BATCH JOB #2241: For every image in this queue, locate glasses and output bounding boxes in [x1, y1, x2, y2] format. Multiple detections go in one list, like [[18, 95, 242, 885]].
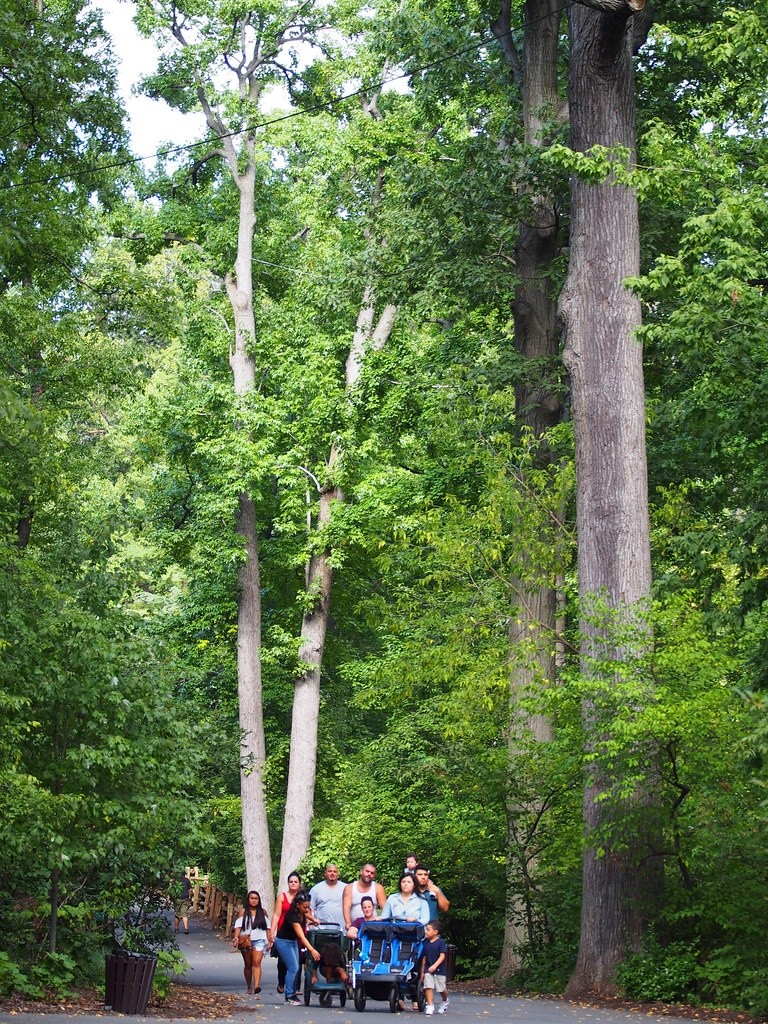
[[297, 894, 311, 901]]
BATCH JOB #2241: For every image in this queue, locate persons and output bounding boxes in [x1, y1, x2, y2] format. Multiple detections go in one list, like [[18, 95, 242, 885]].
[[418, 921, 450, 1015], [232, 890, 273, 994], [269, 854, 451, 1015], [166, 877, 193, 935]]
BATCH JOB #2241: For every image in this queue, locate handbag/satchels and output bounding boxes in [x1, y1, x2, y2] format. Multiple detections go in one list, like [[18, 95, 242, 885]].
[[237, 934, 252, 950]]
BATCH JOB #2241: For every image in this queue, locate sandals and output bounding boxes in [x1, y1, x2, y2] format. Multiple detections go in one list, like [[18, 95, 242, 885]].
[[295, 990, 303, 995], [277, 984, 284, 993]]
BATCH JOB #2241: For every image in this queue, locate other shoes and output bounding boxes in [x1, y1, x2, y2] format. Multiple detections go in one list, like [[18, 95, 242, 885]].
[[429, 892, 438, 900], [398, 1000, 404, 1010], [174, 928, 178, 934], [184, 928, 190, 934], [413, 1002, 418, 1009], [254, 986, 261, 995], [246, 988, 252, 994]]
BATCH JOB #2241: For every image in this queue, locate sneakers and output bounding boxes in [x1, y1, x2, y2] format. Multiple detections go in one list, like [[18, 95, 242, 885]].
[[437, 998, 450, 1014], [284, 994, 302, 1005], [425, 1002, 434, 1014]]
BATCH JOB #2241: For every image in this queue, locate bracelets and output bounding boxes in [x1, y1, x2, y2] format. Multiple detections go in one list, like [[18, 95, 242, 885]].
[[233, 937, 239, 941]]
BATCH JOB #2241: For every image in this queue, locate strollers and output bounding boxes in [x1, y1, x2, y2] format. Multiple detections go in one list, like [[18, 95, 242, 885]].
[[350, 917, 427, 1014], [304, 922, 352, 1009]]
